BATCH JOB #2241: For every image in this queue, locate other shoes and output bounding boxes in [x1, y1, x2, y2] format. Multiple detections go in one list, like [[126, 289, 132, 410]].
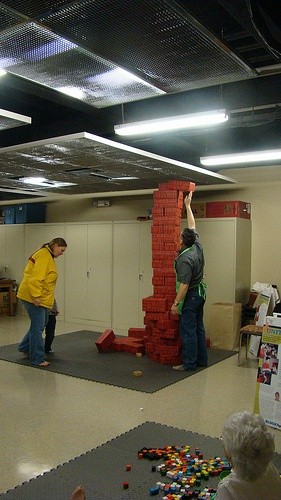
[[173, 365, 185, 370]]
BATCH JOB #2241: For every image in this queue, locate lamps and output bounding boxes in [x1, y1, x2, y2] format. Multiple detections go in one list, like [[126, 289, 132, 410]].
[[112, 109, 281, 167]]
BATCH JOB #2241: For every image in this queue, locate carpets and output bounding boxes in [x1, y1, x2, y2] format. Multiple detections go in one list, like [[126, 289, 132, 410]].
[[0, 421, 281, 500], [3, 330, 239, 394]]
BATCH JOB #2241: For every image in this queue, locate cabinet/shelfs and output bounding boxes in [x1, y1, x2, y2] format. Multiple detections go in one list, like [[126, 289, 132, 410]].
[[0, 216, 253, 328]]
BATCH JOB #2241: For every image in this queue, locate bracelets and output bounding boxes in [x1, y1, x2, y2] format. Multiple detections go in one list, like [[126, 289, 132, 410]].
[[173, 301, 179, 306]]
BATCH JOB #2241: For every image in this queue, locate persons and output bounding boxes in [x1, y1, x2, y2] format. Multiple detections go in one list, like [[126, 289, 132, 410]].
[[45, 300, 59, 354], [170, 191, 208, 372], [16, 238, 67, 367], [216, 411, 281, 500]]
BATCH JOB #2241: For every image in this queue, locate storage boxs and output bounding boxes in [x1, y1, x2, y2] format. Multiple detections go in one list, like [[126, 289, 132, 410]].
[[94, 180, 250, 366], [253, 315, 281, 428]]
